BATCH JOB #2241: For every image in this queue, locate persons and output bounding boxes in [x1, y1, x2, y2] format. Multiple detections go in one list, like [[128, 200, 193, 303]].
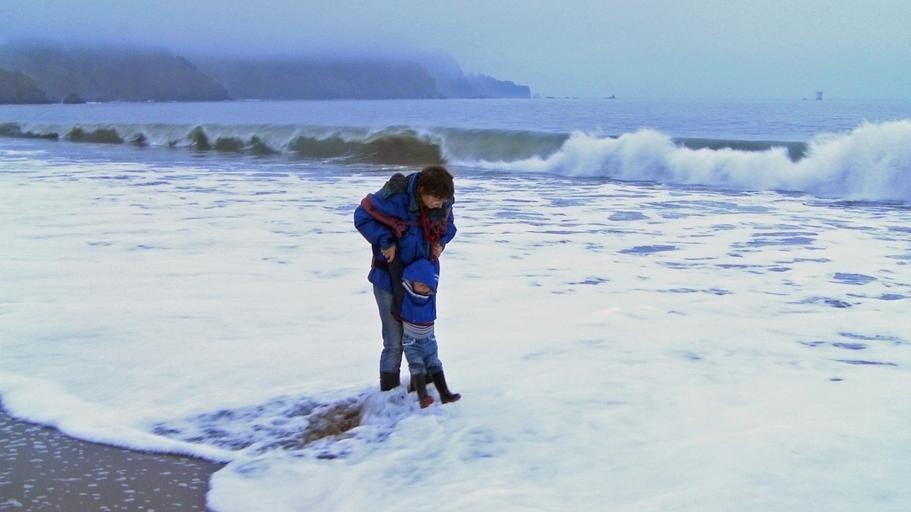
[[387, 245, 461, 409], [354, 167, 457, 391]]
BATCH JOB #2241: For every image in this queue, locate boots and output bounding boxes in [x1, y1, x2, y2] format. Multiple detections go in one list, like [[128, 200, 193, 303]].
[[432, 370, 461, 404], [413, 373, 434, 409]]
